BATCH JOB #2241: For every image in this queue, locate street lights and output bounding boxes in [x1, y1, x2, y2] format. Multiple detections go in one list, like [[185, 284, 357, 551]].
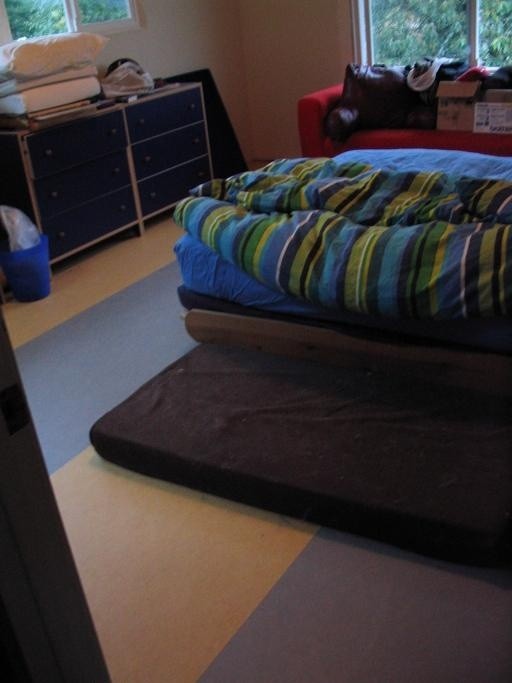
[[342, 64, 404, 112]]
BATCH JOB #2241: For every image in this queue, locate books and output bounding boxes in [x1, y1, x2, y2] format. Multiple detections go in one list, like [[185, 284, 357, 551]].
[[435, 79, 511, 134]]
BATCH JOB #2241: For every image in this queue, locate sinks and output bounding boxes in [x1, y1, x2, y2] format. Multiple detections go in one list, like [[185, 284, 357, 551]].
[[296, 66, 510, 156]]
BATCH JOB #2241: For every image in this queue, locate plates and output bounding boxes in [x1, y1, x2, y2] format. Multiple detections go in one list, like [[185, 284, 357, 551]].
[[0, 78, 214, 274]]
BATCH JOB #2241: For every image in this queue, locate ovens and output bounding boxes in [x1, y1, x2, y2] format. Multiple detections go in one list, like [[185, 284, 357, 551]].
[[171, 148, 511, 380]]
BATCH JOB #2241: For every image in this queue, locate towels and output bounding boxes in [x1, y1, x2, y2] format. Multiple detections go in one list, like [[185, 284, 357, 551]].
[[0, 234, 51, 303]]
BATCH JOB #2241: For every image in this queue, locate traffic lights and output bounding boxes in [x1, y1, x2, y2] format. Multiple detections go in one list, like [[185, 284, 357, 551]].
[[0, 32, 111, 80]]
